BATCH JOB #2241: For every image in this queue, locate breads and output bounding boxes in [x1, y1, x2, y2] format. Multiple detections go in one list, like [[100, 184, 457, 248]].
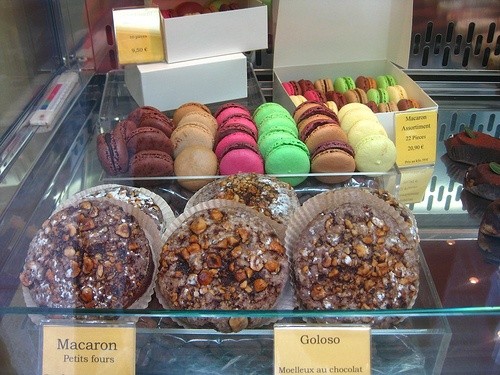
[[444, 126, 500, 250]]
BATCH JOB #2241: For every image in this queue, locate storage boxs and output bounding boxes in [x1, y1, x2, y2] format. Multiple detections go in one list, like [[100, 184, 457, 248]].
[[124, 52, 247, 113], [148, 0, 268, 64], [272, 0, 438, 170]]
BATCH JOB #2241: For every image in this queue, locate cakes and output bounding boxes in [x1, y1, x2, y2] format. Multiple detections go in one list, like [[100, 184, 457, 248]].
[[369, 190, 414, 226], [156, 207, 288, 333], [208, 172, 290, 223], [78, 186, 166, 233], [18, 200, 154, 320], [291, 203, 420, 328]]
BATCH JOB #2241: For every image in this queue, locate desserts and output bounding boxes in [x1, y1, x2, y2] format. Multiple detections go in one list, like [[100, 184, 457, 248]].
[[98, 102, 393, 190], [281, 75, 422, 114]]
